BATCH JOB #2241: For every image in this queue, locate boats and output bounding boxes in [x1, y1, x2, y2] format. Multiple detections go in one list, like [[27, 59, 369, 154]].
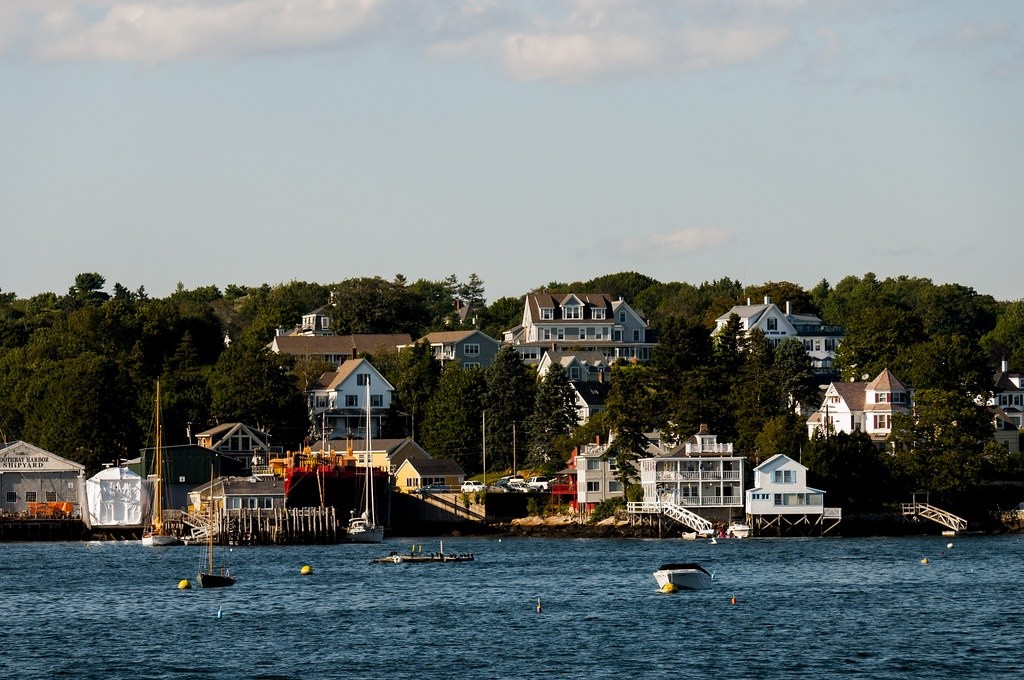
[[725, 524, 752, 540], [370, 554, 475, 564], [683, 531, 707, 539], [652, 562, 716, 592]]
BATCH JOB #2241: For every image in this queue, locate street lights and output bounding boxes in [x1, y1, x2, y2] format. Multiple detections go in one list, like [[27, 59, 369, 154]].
[[483, 408, 492, 486]]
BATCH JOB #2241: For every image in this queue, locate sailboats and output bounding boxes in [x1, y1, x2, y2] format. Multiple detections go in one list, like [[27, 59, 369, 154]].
[[346, 378, 384, 542], [195, 464, 235, 588], [143, 382, 178, 547]]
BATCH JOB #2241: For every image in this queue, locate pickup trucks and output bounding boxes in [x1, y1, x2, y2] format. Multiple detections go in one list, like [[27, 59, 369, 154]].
[[528, 476, 554, 492]]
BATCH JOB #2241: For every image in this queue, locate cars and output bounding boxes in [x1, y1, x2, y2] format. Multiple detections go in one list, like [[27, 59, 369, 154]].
[[461, 480, 487, 493], [508, 482, 531, 493], [416, 482, 451, 493]]
